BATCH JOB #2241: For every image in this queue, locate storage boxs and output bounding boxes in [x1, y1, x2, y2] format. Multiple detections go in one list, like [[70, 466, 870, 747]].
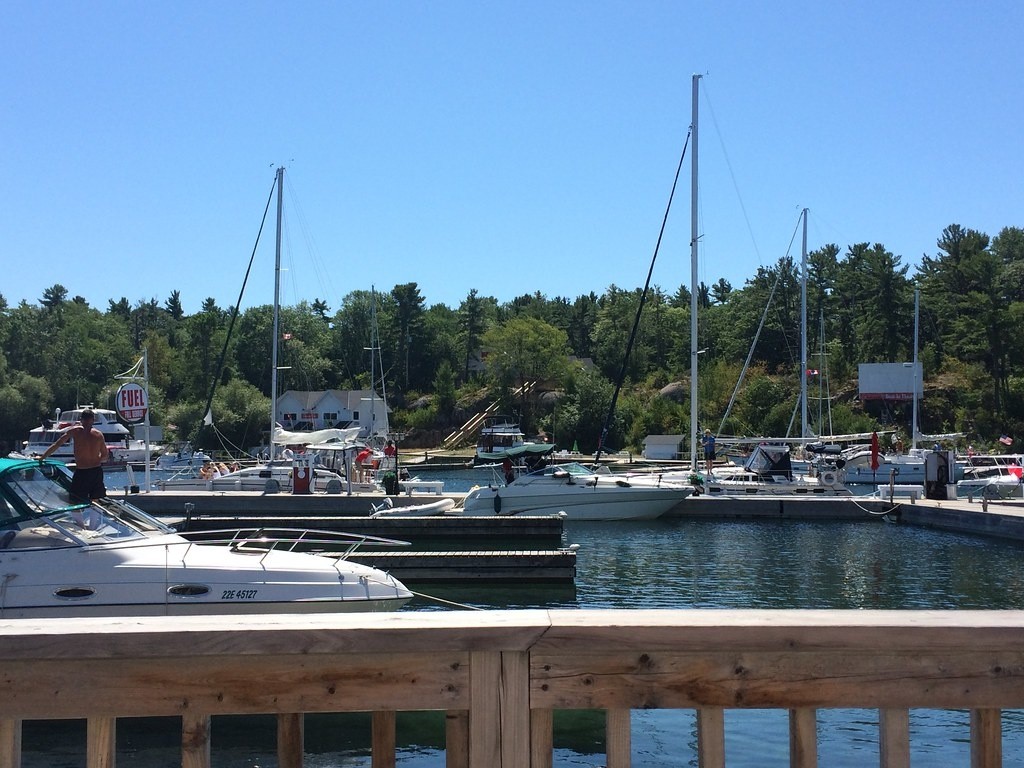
[[878, 484, 924, 500]]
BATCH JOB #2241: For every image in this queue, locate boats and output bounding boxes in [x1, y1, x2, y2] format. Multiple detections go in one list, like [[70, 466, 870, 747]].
[[457, 444, 691, 521], [0, 458, 415, 611], [22, 404, 163, 469]]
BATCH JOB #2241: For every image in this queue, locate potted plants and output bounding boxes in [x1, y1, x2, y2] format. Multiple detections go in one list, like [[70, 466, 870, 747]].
[[687, 474, 705, 496], [380, 470, 397, 495]]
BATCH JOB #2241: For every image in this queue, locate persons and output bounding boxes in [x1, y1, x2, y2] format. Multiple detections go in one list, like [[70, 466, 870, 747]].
[[897, 436, 904, 458], [933, 440, 942, 453], [526, 450, 548, 476], [968, 442, 974, 459], [33, 410, 110, 531], [355, 439, 400, 488], [702, 428, 717, 476], [504, 454, 517, 486], [198, 459, 240, 483]]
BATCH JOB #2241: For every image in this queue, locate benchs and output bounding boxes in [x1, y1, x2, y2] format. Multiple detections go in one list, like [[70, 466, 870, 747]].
[[402, 481, 445, 496]]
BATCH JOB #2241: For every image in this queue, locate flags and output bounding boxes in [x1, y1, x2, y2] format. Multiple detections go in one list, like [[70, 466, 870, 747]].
[[204, 408, 213, 426], [807, 368, 821, 376], [1000, 433, 1015, 445]]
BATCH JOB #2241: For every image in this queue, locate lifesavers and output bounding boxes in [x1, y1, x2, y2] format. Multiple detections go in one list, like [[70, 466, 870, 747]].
[[821, 471, 837, 486]]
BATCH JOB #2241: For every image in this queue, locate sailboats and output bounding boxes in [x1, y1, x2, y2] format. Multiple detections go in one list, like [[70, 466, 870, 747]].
[[104, 159, 466, 516], [584, 72, 829, 495], [701, 199, 1024, 495]]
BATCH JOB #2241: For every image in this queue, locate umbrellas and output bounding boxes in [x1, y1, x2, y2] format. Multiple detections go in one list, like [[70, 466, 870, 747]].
[[871, 429, 881, 494]]
